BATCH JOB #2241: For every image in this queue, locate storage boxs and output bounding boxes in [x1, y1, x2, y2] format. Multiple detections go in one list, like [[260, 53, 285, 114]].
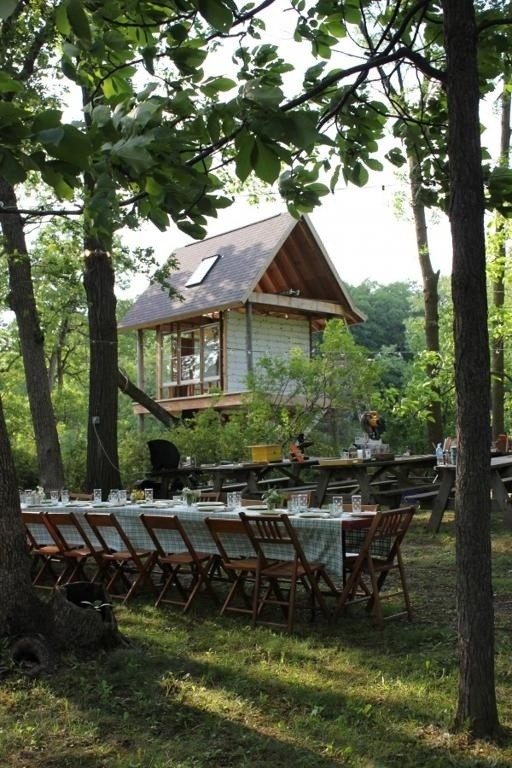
[[247, 443, 283, 462]]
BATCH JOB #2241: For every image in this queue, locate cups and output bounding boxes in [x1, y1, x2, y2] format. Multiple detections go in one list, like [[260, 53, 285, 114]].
[[332, 495, 362, 516], [227, 491, 242, 509], [287, 494, 308, 513], [26, 488, 182, 508], [340, 449, 371, 460]]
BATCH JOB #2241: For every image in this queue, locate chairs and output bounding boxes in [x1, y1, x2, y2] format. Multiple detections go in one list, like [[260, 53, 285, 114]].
[[20, 483, 417, 631], [136, 436, 181, 496]]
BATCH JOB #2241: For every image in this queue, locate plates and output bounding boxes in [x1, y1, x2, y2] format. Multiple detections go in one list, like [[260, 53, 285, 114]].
[[197, 502, 226, 512], [301, 512, 329, 518], [247, 502, 283, 515], [350, 512, 379, 516]]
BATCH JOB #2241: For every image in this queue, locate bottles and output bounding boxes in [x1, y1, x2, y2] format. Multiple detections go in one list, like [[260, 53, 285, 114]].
[[436, 443, 449, 465]]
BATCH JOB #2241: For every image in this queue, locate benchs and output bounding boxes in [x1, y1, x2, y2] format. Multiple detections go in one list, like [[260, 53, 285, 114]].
[[148, 456, 510, 533]]
